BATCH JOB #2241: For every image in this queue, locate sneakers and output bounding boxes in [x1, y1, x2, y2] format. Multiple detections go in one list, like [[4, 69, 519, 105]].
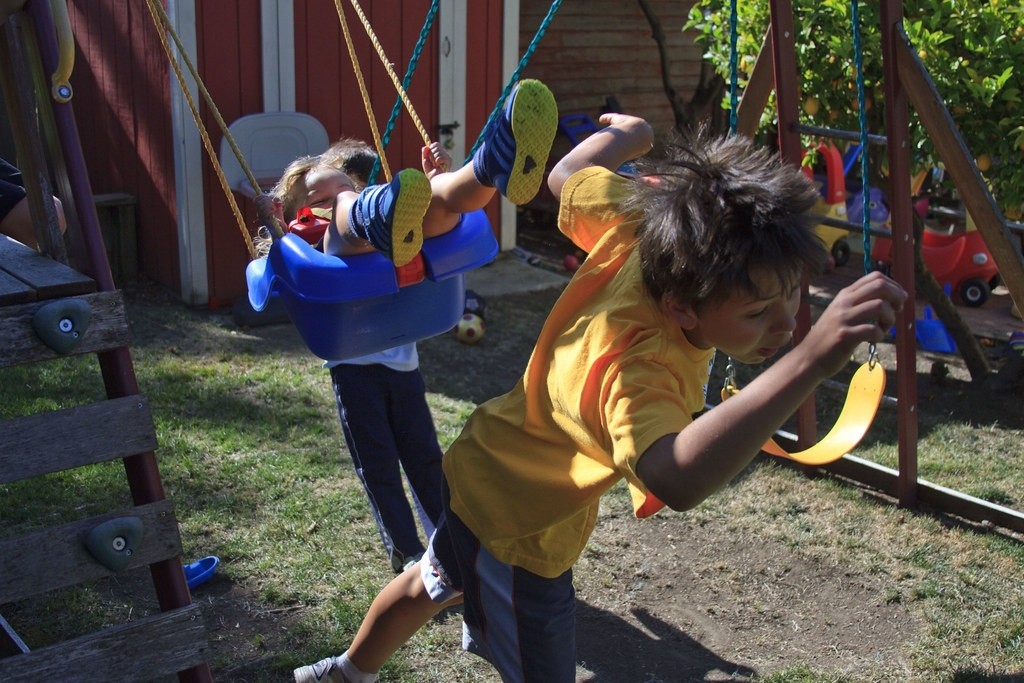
[[361, 168, 433, 269], [294, 654, 346, 683], [483, 79, 559, 206]]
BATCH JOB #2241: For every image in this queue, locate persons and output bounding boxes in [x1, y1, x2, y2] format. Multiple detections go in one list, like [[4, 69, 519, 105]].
[[0, 148, 66, 252], [322, 137, 453, 575], [292, 113, 907, 683], [252, 78, 557, 267]]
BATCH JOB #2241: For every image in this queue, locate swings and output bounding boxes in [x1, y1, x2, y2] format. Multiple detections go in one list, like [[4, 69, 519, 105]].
[[145, 0, 501, 362], [719, 0, 888, 466]]
